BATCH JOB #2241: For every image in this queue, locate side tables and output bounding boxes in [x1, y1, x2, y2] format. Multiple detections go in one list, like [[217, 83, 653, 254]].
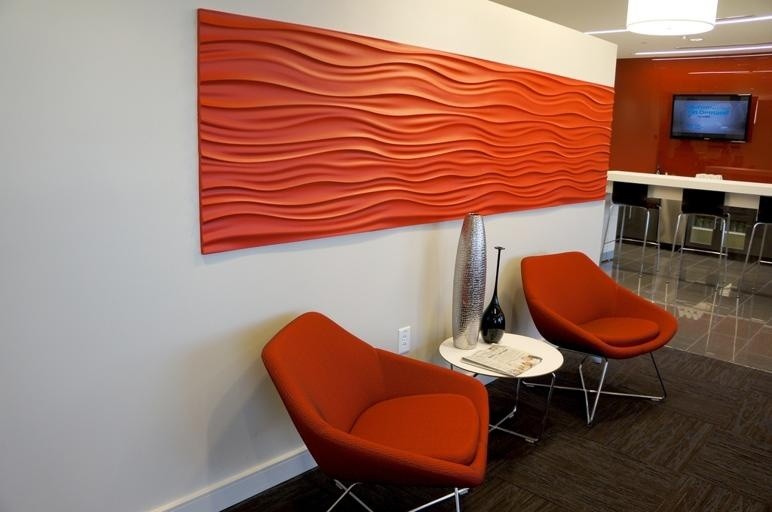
[[438, 331, 565, 442]]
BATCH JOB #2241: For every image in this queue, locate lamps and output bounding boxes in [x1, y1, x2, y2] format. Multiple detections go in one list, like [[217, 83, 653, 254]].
[[625, 0, 719, 37]]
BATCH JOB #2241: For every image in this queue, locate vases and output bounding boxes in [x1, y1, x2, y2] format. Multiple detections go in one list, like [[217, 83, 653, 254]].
[[481, 247, 506, 344], [452, 213, 487, 350]]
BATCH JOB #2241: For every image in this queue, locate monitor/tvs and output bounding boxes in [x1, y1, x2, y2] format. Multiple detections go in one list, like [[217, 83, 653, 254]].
[[669, 93, 752, 143]]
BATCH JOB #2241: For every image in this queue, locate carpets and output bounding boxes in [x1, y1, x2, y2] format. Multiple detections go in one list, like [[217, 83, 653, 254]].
[[216, 346, 772, 512]]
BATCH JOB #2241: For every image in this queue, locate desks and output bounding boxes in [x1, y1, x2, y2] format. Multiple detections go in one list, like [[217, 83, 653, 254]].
[[600, 170, 772, 263]]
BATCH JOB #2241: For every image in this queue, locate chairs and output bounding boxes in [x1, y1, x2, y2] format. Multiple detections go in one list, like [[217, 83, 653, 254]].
[[520, 251, 678, 425], [261, 312, 490, 512], [736, 196, 772, 299], [600, 181, 662, 278], [666, 188, 731, 292]]
